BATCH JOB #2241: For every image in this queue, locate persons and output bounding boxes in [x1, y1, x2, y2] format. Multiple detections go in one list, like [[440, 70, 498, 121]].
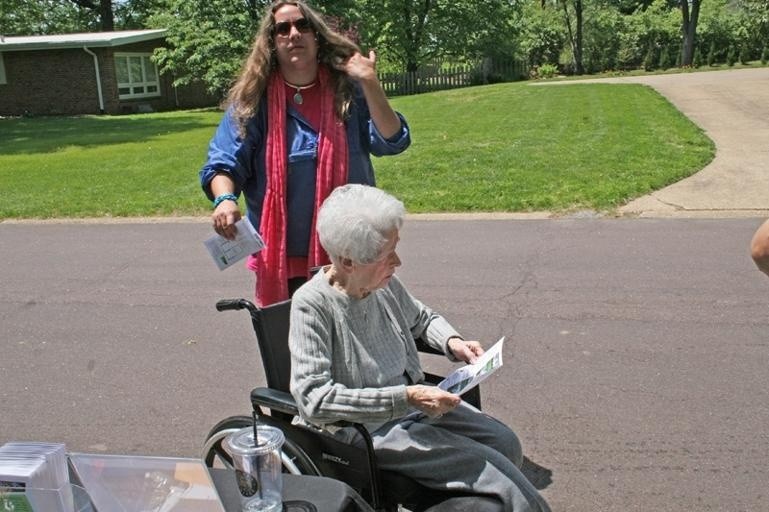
[[286, 182, 550, 510], [747, 218, 769, 281], [201, 1, 410, 307]]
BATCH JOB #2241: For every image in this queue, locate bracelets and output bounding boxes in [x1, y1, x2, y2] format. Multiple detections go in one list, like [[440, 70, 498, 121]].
[[212, 193, 238, 208]]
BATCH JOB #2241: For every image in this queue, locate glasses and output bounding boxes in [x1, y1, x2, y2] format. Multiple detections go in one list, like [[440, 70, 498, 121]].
[[273, 16, 313, 37]]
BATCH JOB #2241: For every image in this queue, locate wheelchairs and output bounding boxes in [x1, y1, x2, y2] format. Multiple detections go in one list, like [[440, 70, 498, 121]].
[[200, 265, 482, 512]]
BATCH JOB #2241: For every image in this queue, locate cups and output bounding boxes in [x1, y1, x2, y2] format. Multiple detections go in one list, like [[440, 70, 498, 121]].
[[225, 423, 288, 512]]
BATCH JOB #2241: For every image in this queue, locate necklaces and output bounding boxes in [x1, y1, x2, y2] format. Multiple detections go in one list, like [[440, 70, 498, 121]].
[[286, 82, 318, 104]]
